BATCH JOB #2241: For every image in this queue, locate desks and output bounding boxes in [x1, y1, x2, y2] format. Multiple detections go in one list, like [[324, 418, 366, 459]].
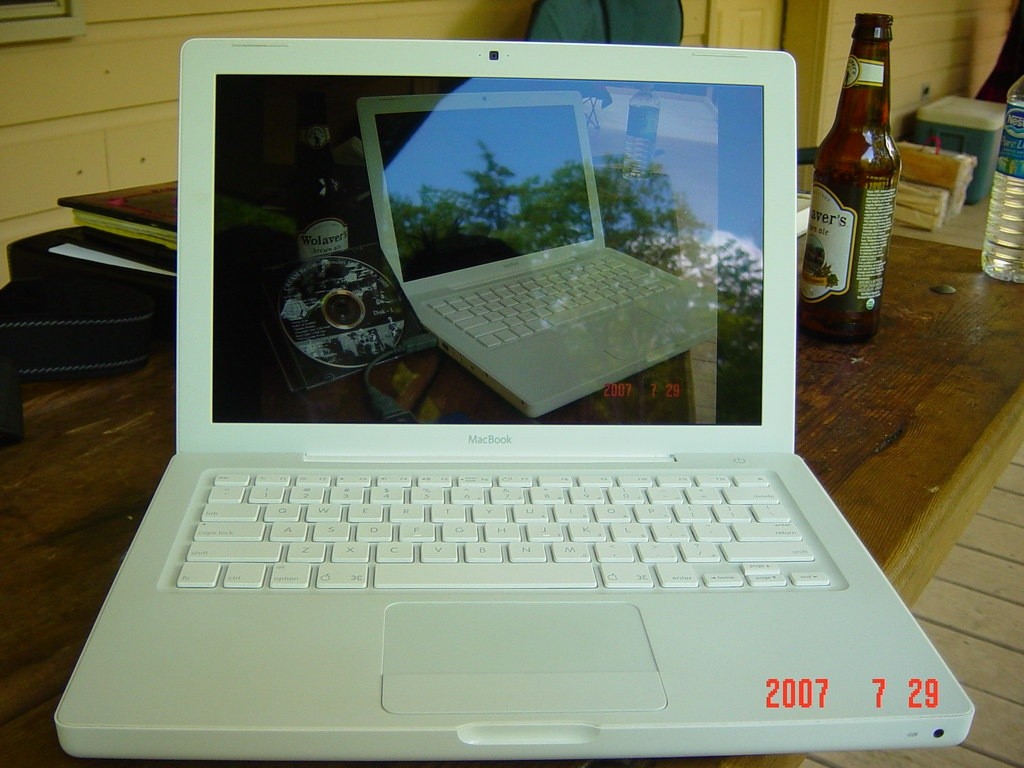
[[1, 236, 1024, 768]]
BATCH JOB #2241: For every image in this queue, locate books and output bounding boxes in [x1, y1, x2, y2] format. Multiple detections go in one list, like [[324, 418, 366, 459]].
[[57, 180, 178, 251]]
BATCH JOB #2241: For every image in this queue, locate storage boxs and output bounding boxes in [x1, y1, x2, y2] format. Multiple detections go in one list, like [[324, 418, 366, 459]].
[[892, 95, 1007, 232]]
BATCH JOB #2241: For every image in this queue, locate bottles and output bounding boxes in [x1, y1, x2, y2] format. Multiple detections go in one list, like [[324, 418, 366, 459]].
[[296, 91, 351, 259], [623, 85, 660, 181], [798, 13, 901, 343], [981, 72, 1024, 284]]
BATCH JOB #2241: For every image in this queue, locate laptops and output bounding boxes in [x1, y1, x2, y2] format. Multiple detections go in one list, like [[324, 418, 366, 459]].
[[55, 37, 976, 763], [357, 91, 719, 419]]
